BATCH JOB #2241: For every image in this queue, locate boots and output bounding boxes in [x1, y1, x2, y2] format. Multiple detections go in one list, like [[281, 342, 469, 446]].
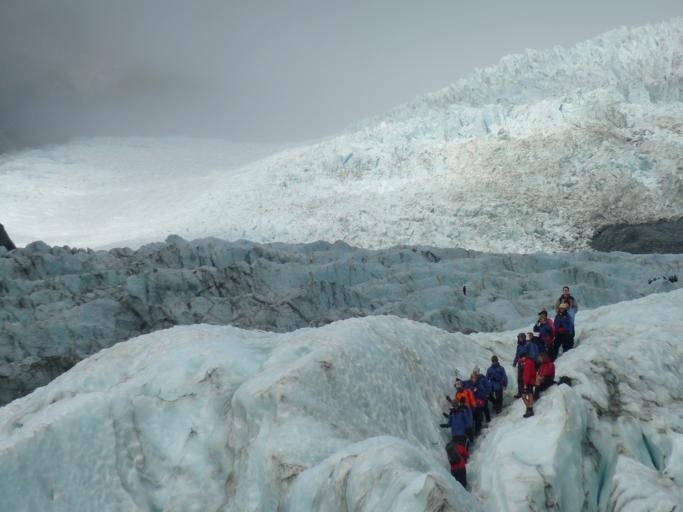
[[524, 407, 534, 417]]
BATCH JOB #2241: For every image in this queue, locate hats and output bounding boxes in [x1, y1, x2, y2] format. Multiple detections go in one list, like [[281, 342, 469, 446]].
[[559, 304, 567, 311], [473, 368, 480, 376], [455, 381, 462, 388], [452, 399, 458, 406], [539, 311, 547, 315]]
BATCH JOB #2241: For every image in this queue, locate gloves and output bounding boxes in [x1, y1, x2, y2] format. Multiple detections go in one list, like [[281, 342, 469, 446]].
[[440, 424, 447, 428], [443, 412, 448, 418], [446, 396, 451, 402]]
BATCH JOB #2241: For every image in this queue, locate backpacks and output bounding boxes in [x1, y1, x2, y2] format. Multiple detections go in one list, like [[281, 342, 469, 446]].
[[445, 443, 461, 465]]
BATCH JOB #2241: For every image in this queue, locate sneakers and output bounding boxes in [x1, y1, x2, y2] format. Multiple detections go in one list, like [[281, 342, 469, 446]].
[[514, 392, 521, 398], [474, 402, 502, 435]]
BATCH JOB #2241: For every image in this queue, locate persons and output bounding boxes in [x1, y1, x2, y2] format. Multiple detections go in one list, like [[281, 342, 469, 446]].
[[437, 287, 577, 488]]
